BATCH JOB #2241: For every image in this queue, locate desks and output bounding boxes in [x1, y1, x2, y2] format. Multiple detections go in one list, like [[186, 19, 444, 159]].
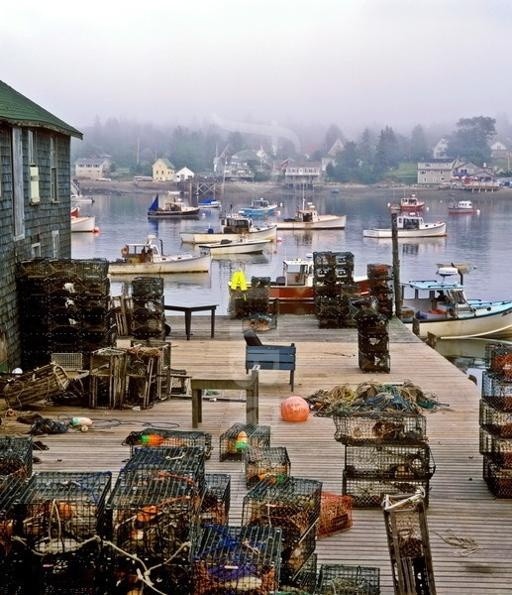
[[164, 305, 218, 340]]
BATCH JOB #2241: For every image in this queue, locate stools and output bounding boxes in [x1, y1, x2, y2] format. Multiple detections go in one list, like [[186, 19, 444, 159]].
[[191, 365, 260, 427]]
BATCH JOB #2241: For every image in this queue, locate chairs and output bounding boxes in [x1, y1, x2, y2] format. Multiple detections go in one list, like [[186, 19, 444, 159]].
[[245, 343, 296, 392]]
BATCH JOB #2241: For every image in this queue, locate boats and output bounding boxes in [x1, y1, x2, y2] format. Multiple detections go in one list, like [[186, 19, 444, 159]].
[[70, 188, 96, 234]]
